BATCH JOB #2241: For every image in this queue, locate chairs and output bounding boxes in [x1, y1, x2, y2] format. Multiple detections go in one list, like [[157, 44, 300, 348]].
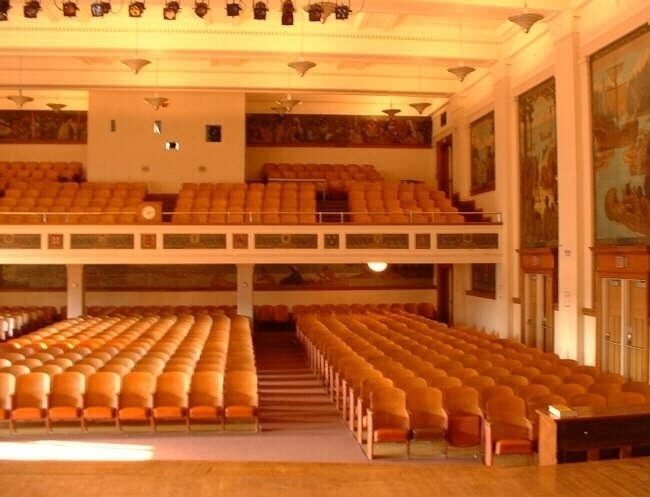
[[0, 161, 147, 223], [347, 179, 464, 226], [170, 180, 317, 226], [0, 302, 262, 435], [292, 301, 650, 464], [252, 304, 295, 332], [262, 162, 384, 198]]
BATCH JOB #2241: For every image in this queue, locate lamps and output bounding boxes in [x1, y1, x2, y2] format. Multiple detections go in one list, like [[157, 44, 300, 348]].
[[225, 2, 241, 15], [271, 104, 288, 119], [281, 94, 300, 112], [280, 1, 295, 25], [121, 57, 151, 75], [205, 124, 222, 143], [162, 1, 181, 20], [448, 62, 476, 83], [381, 101, 401, 119], [151, 103, 169, 111], [288, 54, 317, 78], [192, 2, 210, 17], [144, 97, 168, 104], [46, 103, 67, 112], [333, 1, 351, 19], [253, 1, 268, 20], [62, 2, 79, 17], [128, 2, 147, 17], [508, 1, 544, 33], [90, 1, 113, 17], [409, 102, 432, 115], [24, 1, 41, 16], [4, 56, 34, 107], [307, 1, 323, 22]]
